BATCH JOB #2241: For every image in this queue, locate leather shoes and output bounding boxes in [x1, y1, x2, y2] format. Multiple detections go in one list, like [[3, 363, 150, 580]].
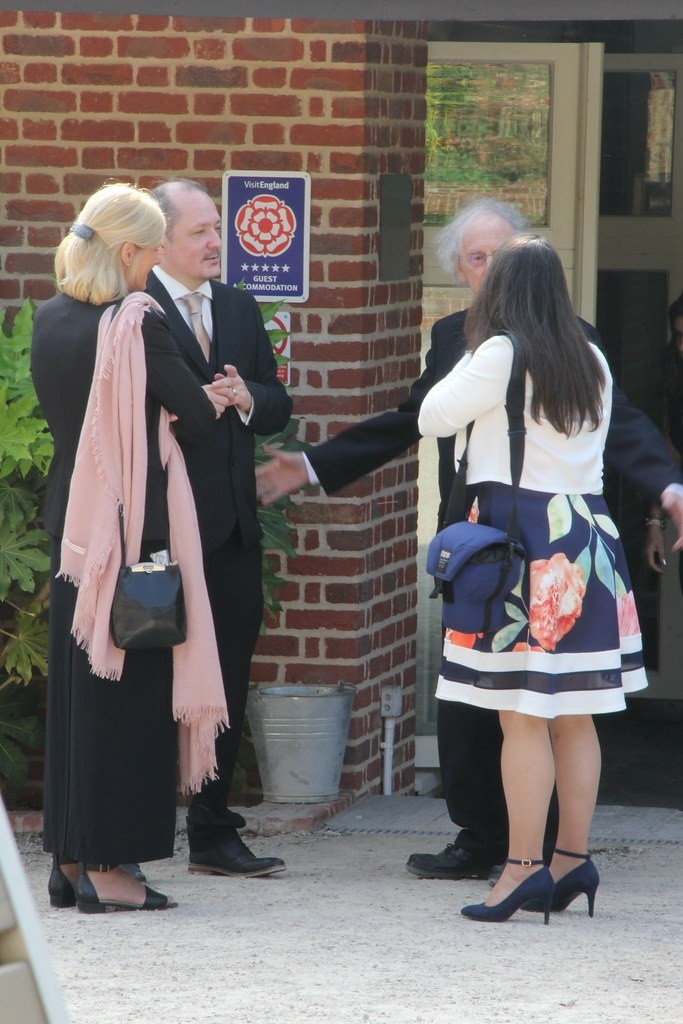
[[187, 834, 286, 878], [120, 863, 147, 882], [406, 830, 491, 879]]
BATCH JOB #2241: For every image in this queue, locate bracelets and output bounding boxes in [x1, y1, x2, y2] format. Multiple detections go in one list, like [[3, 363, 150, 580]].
[[644, 512, 666, 529]]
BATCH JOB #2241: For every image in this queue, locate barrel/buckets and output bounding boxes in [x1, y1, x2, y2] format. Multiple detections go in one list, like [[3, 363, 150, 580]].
[[246, 680, 357, 803]]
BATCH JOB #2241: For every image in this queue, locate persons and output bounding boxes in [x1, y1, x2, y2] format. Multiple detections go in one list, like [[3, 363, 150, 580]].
[[256, 200, 683, 881], [418, 235, 648, 925], [153, 176, 295, 879], [644, 294, 682, 592], [31, 183, 219, 913]]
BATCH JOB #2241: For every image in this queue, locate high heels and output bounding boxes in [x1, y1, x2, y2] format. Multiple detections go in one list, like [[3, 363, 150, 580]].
[[522, 849, 600, 918], [460, 860, 554, 926]]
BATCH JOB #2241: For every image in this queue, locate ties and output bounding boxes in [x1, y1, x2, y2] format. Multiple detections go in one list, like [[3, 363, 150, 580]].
[[181, 294, 210, 363]]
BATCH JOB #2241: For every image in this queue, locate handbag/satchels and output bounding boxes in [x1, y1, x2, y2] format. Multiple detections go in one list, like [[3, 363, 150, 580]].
[[110, 562, 187, 650], [427, 522, 524, 633]]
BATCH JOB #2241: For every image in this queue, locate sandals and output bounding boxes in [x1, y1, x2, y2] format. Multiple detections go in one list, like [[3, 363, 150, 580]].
[[77, 861, 178, 910], [49, 854, 77, 908]]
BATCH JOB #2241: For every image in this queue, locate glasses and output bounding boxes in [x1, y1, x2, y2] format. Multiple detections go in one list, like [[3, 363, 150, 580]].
[[467, 251, 501, 267]]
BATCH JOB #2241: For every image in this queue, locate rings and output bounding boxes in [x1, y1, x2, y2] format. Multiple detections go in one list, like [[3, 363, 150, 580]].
[[231, 388, 236, 394]]
